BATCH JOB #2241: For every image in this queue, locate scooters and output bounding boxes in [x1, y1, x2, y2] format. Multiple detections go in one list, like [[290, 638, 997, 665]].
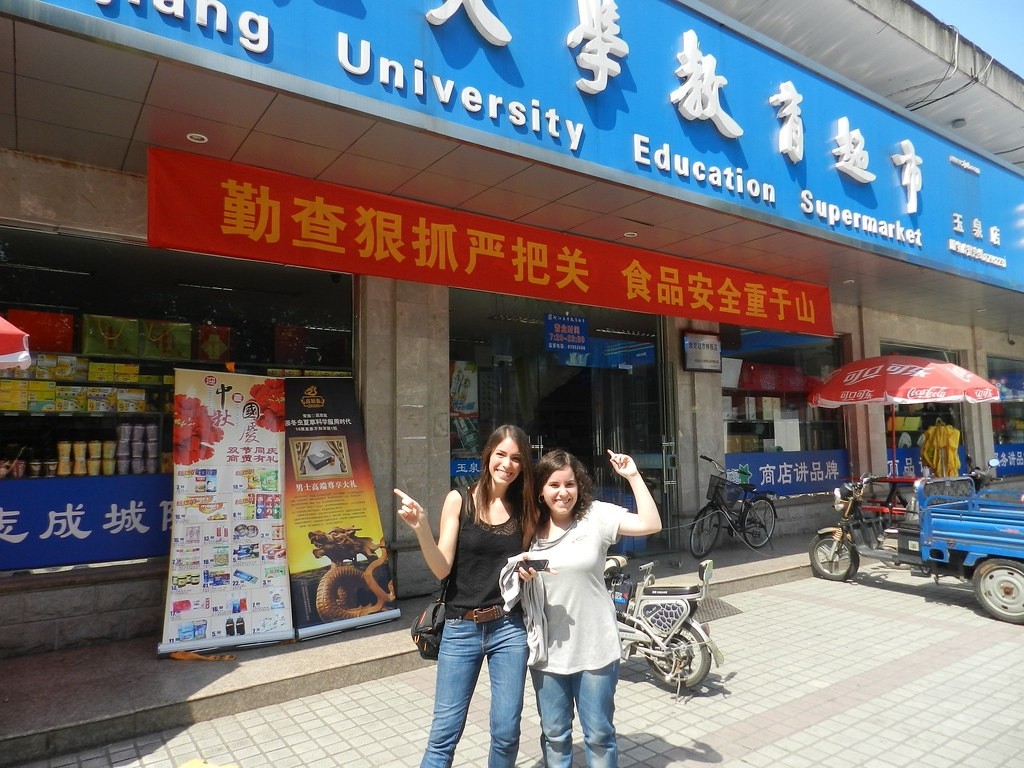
[[603, 554, 726, 703]]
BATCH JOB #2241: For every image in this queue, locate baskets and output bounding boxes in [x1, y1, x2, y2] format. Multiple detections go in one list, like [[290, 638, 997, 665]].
[[706, 474, 744, 509]]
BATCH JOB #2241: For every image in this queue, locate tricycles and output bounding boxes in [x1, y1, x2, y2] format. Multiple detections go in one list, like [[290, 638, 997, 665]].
[[809, 461, 1024, 627]]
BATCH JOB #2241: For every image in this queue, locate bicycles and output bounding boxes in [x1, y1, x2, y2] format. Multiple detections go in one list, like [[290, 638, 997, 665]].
[[690, 454, 779, 559]]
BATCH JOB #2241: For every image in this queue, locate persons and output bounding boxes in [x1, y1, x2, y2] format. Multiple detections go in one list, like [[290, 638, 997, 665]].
[[519, 448, 662, 768], [391, 425, 537, 768]]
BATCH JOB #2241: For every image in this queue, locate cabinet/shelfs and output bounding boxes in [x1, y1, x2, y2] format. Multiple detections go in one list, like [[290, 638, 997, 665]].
[[722, 386, 838, 436], [992, 401, 1024, 432], [0, 350, 353, 474], [885, 410, 961, 438]]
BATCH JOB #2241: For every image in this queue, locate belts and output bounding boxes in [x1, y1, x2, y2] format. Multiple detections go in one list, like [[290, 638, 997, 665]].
[[461, 604, 522, 624]]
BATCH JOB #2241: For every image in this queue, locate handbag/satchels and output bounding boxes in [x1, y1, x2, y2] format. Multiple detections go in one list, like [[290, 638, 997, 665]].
[[410, 603, 446, 661]]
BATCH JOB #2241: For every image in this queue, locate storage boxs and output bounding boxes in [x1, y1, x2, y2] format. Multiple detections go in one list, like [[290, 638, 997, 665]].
[[114, 374, 139, 382], [138, 375, 163, 384], [162, 389, 174, 403], [193, 325, 231, 362], [144, 391, 163, 402], [722, 358, 829, 454], [0, 353, 118, 413], [138, 318, 191, 359], [163, 375, 174, 384], [117, 400, 149, 412], [5, 309, 74, 353], [118, 388, 149, 400], [76, 314, 139, 357], [274, 325, 306, 364], [115, 363, 140, 374], [163, 403, 174, 413], [148, 402, 163, 412]]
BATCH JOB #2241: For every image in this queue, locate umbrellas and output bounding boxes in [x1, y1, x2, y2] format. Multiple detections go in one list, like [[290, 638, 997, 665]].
[[806, 353, 1001, 477]]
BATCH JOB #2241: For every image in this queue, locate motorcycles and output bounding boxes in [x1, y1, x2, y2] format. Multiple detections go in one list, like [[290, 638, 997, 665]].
[[961, 453, 1004, 496]]
